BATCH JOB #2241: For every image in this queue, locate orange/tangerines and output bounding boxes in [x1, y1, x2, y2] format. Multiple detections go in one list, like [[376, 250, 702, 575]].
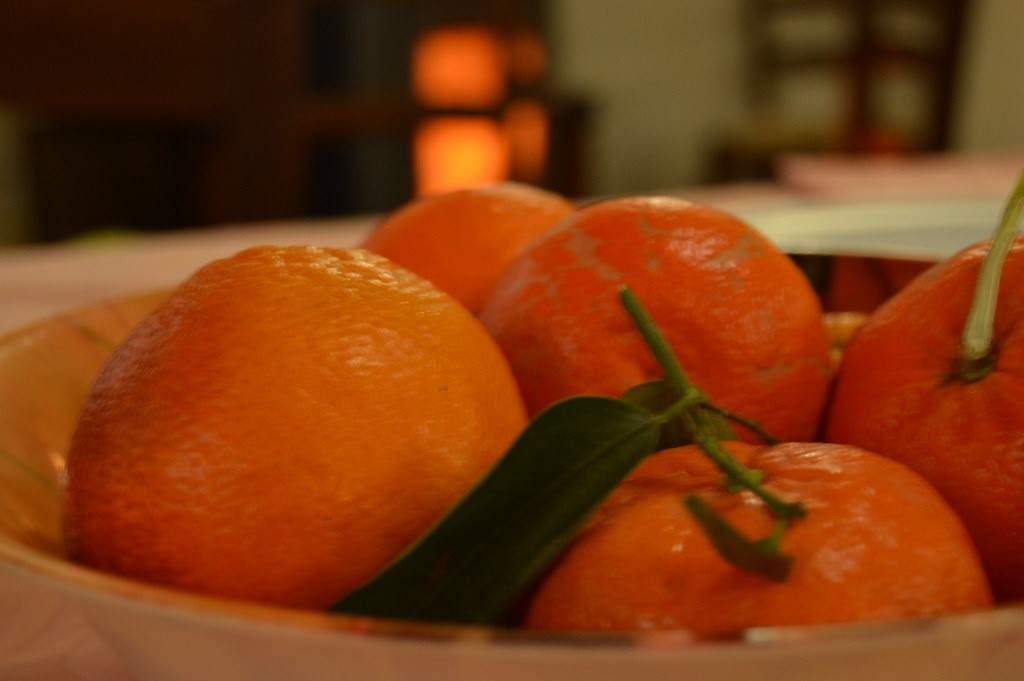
[[62, 182, 1024, 627]]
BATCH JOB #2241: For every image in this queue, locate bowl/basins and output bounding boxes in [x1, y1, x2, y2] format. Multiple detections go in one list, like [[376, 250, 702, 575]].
[[0, 252, 1024, 681]]
